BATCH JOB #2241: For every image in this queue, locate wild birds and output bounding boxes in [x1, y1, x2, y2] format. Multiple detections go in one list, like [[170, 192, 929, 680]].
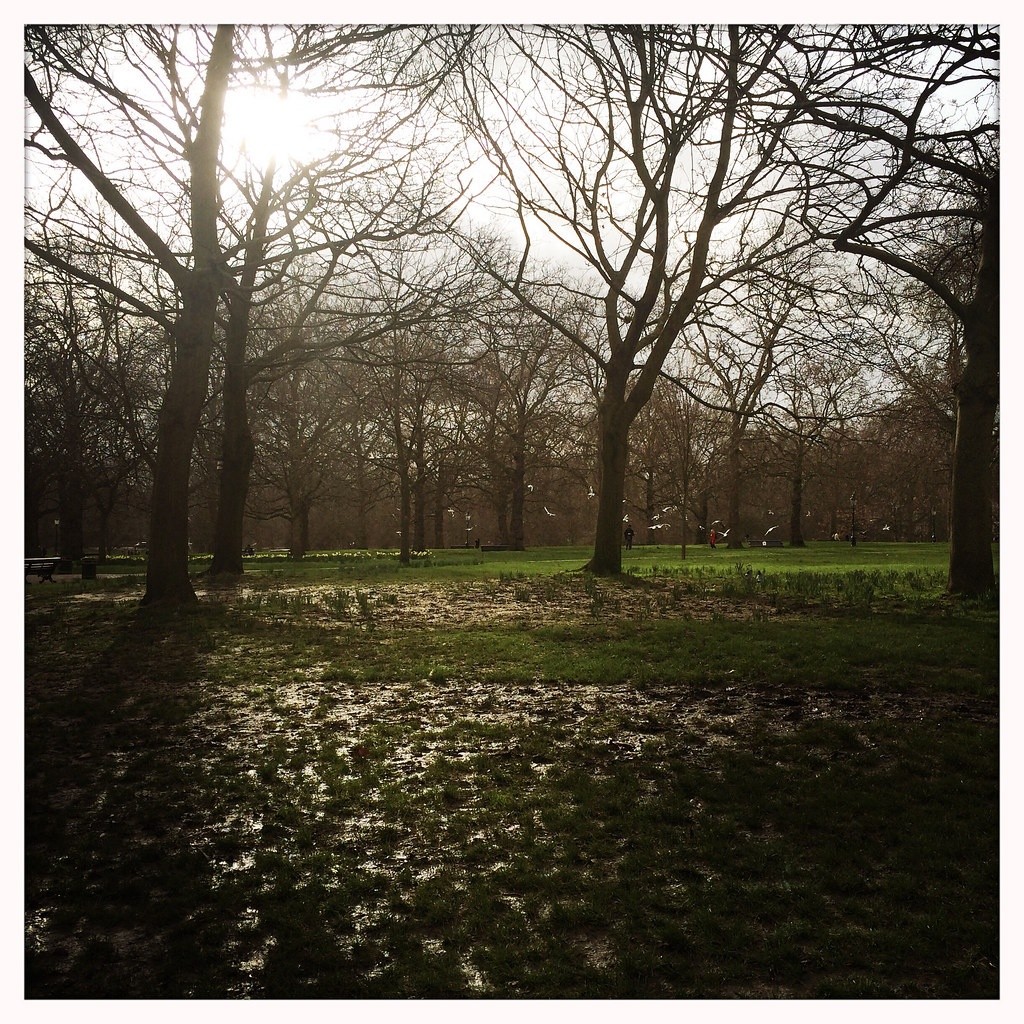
[[882, 524, 890, 530], [806, 510, 811, 516], [768, 510, 774, 515], [648, 506, 671, 529], [623, 514, 629, 523], [587, 485, 595, 498], [718, 529, 731, 538], [544, 507, 556, 516], [764, 525, 779, 535]]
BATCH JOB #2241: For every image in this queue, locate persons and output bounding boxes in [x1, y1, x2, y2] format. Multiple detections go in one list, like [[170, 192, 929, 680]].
[[475, 538, 479, 548], [624, 525, 634, 550], [245, 545, 253, 556], [709, 529, 716, 548]]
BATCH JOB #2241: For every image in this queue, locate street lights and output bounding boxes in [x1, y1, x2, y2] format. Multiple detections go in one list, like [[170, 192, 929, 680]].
[[465, 512, 471, 548], [850, 491, 857, 547], [54, 515, 61, 557], [931, 505, 936, 543]]
